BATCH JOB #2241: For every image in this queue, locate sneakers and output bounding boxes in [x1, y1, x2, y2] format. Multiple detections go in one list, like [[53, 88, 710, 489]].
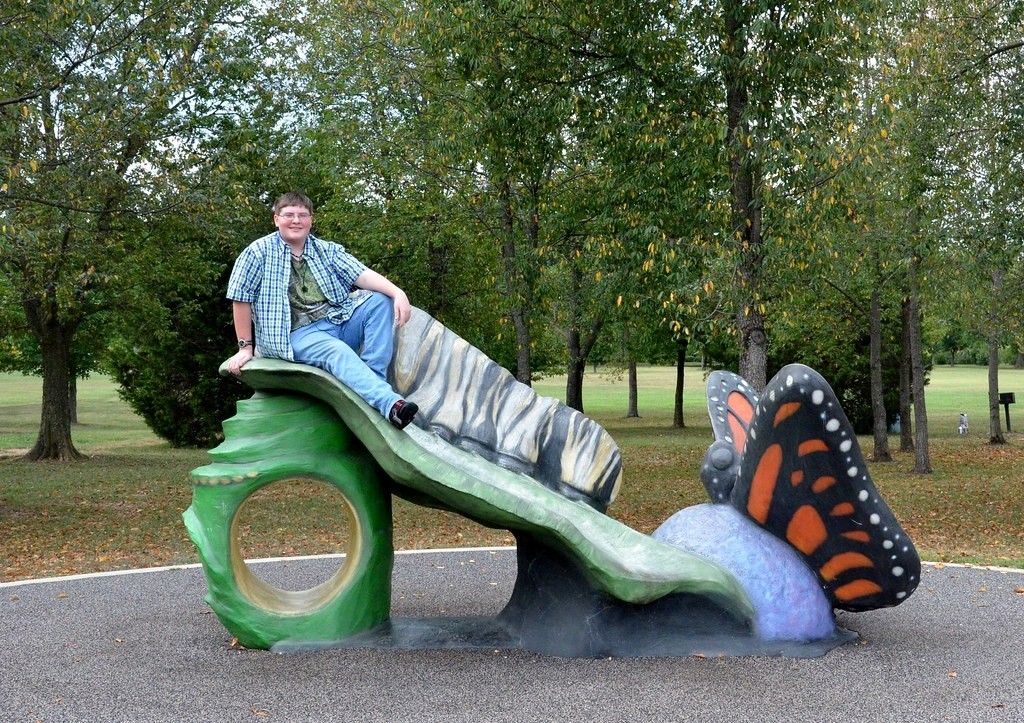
[[389, 400, 419, 430]]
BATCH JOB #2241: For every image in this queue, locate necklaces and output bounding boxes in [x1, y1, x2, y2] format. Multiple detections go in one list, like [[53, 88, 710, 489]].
[[290, 251, 313, 293]]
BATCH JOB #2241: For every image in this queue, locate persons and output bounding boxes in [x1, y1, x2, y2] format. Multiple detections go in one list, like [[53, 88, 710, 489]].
[[228, 192, 419, 430]]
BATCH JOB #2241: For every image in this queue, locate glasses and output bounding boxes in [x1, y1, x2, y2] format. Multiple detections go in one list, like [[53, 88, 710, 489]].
[[277, 212, 312, 221]]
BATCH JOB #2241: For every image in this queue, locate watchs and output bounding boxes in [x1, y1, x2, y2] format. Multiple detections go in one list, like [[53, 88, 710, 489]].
[[237, 338, 253, 348]]
